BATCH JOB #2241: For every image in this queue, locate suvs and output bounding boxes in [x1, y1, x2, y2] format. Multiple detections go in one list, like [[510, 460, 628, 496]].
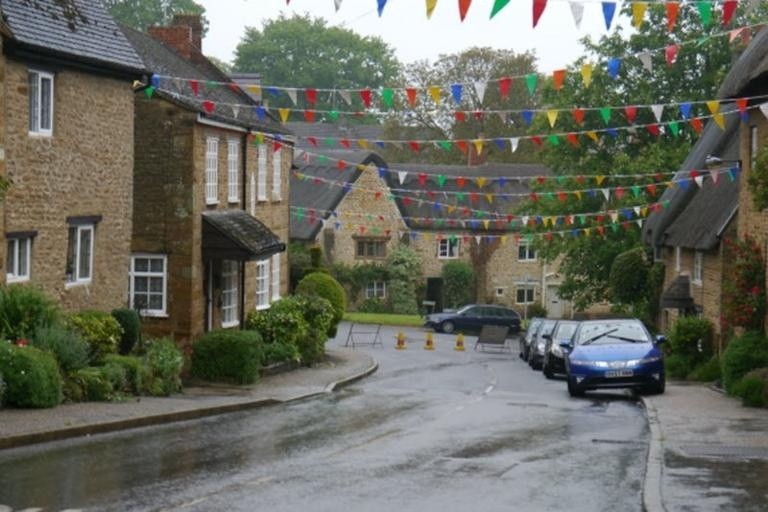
[[424, 305, 521, 340]]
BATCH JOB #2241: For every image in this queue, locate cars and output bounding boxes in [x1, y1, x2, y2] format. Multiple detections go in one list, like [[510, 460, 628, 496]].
[[517, 315, 668, 398]]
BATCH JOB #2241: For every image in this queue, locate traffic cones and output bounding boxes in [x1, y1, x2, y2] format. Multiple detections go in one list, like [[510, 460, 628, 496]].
[[395, 330, 408, 350], [452, 332, 464, 351], [424, 332, 436, 350]]
